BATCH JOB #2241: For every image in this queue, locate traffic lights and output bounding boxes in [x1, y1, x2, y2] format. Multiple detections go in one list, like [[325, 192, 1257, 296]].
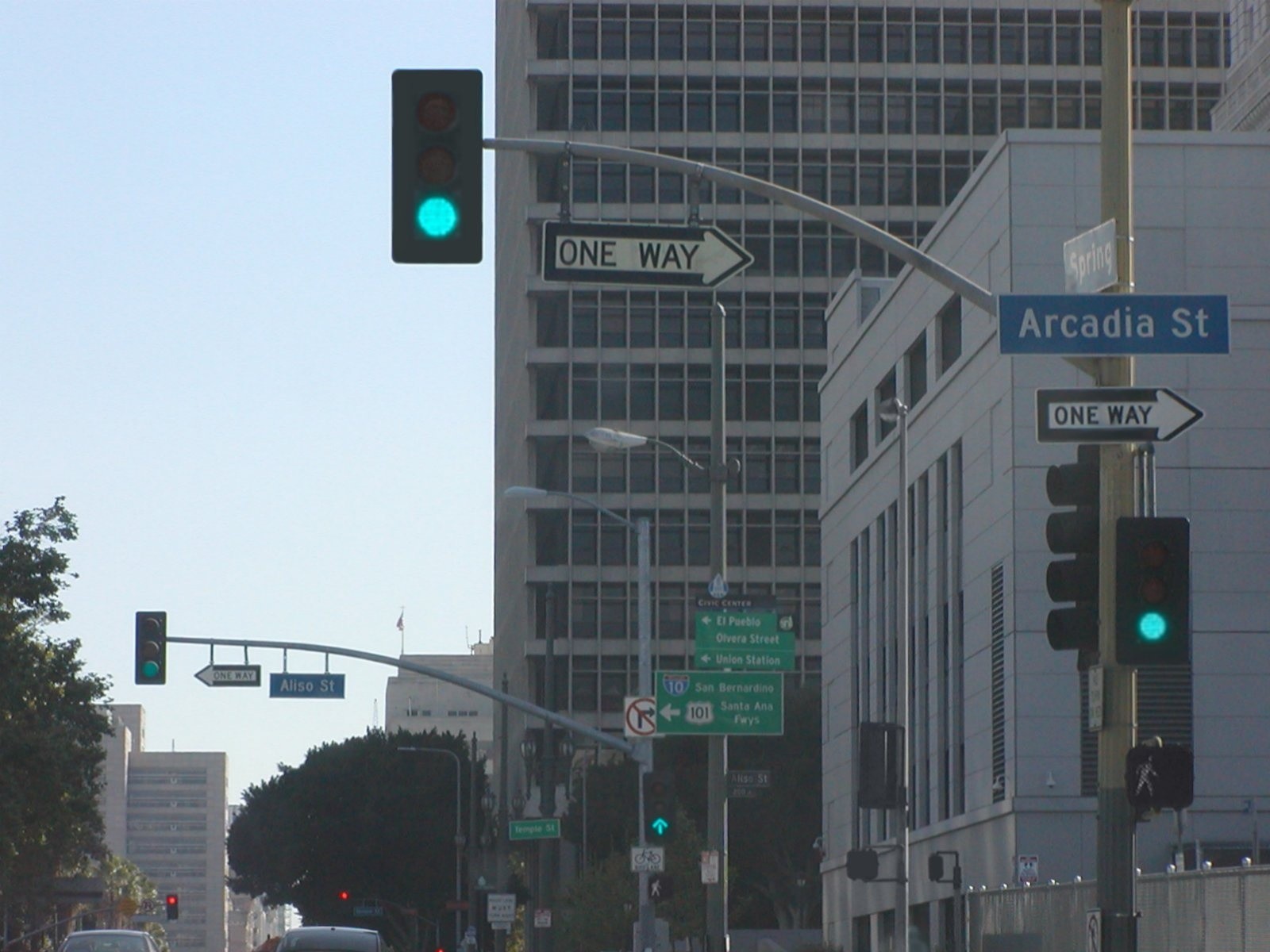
[[1043, 436, 1101, 669], [335, 888, 349, 910], [134, 611, 167, 685], [390, 68, 484, 265], [165, 894, 179, 920], [1125, 735, 1195, 814], [1113, 516, 1193, 668]]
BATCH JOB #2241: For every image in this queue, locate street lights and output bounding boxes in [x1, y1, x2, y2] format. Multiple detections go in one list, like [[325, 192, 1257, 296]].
[[583, 425, 730, 952], [503, 482, 656, 952], [397, 746, 463, 951]]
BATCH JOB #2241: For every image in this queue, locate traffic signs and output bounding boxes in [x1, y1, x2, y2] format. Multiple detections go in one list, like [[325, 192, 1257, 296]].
[[540, 218, 755, 288], [195, 663, 262, 687], [1035, 388, 1206, 443]]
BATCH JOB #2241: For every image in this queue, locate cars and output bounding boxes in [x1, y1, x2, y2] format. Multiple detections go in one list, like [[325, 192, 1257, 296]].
[[276, 925, 390, 952], [57, 929, 169, 952]]
